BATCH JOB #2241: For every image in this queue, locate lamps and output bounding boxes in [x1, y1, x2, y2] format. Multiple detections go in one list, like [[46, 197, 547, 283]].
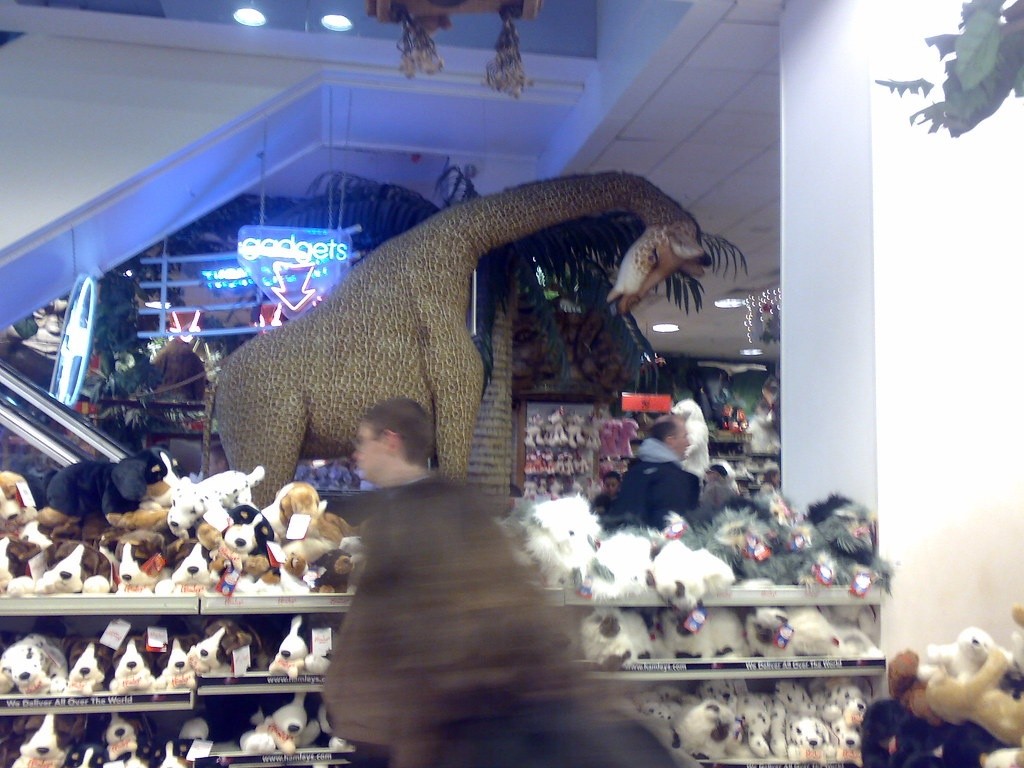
[[317, 1, 355, 33], [231, 0, 270, 26]]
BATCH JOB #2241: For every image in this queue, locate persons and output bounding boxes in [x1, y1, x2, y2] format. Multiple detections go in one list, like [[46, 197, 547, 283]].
[[611, 415, 699, 532], [592, 471, 621, 513], [700, 465, 736, 508], [318, 397, 504, 768], [759, 469, 779, 496], [151, 323, 206, 432]]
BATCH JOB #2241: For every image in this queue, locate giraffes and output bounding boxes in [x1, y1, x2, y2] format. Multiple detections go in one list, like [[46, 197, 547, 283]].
[[218, 170, 711, 511]]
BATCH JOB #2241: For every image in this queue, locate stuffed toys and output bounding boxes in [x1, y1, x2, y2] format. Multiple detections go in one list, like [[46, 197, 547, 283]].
[[215, 168, 782, 511], [0, 451, 1024, 768]]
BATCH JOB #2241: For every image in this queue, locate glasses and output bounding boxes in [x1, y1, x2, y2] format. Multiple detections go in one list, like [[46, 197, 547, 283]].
[[352, 435, 378, 448]]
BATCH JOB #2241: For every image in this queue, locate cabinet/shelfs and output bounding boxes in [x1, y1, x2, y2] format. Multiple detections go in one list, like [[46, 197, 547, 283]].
[[0, 447, 194, 768], [196, 465, 884, 768]]
